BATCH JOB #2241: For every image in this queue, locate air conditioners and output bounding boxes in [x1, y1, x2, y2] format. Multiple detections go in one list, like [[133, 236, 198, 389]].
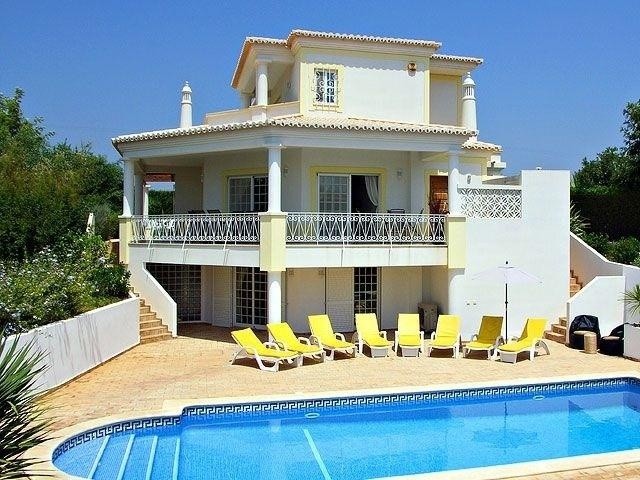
[[416, 302, 438, 333]]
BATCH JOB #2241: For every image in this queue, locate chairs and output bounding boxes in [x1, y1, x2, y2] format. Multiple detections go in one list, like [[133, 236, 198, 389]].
[[266, 322, 326, 367], [351, 312, 394, 358], [465, 316, 507, 360], [229, 327, 298, 373], [498, 318, 550, 364], [429, 315, 464, 360], [393, 315, 424, 358], [306, 314, 358, 361]]
